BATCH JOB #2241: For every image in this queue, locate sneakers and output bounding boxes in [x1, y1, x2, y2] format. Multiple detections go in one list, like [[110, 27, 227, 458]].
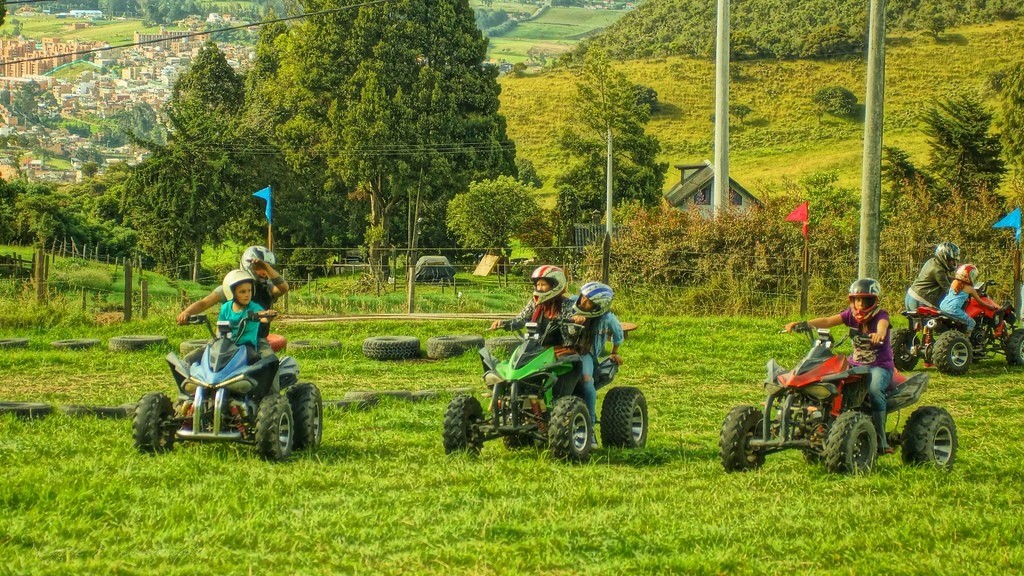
[[589, 428, 599, 450]]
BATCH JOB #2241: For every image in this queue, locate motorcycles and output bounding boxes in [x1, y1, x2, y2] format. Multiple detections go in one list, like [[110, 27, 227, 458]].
[[891, 280, 1024, 377], [442, 319, 648, 462], [132, 312, 323, 463], [718, 321, 959, 475]]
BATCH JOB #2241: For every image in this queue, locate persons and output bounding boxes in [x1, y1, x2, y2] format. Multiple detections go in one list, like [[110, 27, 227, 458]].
[[902, 241, 996, 340], [783, 278, 895, 455], [488, 264, 625, 452], [175, 245, 290, 435]]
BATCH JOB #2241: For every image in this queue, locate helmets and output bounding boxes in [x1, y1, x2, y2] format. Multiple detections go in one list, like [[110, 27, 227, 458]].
[[954, 263, 982, 287], [848, 278, 884, 324], [573, 281, 614, 319], [530, 265, 566, 305], [223, 269, 257, 301], [240, 245, 277, 281], [933, 242, 961, 271]]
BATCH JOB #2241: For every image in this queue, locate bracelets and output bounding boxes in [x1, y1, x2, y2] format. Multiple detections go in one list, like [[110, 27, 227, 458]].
[[271, 274, 286, 286]]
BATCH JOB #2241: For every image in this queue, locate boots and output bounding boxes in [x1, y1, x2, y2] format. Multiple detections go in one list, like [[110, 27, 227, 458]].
[[871, 410, 892, 454]]
